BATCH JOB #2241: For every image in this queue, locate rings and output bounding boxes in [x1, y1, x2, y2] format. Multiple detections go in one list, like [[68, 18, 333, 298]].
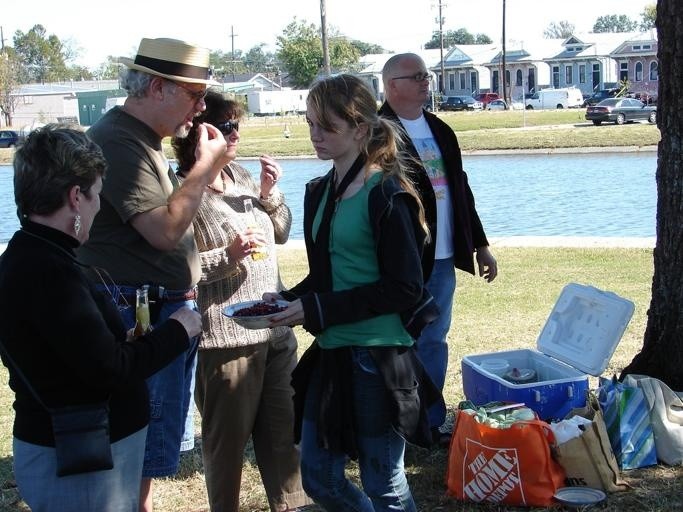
[[288, 325, 295, 328], [243, 241, 250, 250]]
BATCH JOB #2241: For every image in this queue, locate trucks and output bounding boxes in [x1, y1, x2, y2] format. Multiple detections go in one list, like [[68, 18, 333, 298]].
[[524, 88, 584, 110], [245, 89, 312, 117], [104, 96, 127, 114]]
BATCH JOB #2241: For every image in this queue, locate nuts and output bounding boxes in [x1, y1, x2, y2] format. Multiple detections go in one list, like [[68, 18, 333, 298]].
[[232, 302, 288, 316]]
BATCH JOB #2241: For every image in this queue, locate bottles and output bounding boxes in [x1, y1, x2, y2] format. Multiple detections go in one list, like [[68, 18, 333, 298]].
[[130, 285, 153, 336], [242, 199, 269, 262]]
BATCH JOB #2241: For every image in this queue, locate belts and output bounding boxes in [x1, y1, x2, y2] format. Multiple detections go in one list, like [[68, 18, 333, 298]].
[[106, 286, 201, 309]]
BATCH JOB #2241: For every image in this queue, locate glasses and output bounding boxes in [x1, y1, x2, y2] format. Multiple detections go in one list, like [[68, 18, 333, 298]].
[[218, 121, 238, 137], [394, 73, 432, 82], [176, 86, 207, 100]]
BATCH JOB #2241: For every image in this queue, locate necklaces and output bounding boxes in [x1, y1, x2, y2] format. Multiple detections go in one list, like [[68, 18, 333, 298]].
[[206, 171, 226, 194]]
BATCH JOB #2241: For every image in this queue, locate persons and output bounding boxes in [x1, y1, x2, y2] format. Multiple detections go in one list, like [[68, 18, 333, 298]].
[[74, 39, 228, 512], [0, 124, 202, 512], [376, 53, 497, 450], [173, 91, 311, 512], [262, 75, 440, 512]]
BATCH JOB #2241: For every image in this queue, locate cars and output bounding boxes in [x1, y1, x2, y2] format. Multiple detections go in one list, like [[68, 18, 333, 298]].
[[583, 88, 620, 107], [584, 98, 657, 125], [486, 98, 525, 112], [440, 96, 476, 111], [624, 92, 652, 104], [0, 130, 24, 149]]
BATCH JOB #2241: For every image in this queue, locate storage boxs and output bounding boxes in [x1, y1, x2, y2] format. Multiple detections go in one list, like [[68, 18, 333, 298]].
[[461, 283, 635, 423]]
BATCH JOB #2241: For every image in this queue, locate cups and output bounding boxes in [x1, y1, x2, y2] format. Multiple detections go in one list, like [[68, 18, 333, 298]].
[[505, 367, 538, 384], [478, 358, 510, 376]]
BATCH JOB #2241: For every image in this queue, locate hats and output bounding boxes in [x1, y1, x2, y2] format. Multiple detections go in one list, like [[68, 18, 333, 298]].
[[115, 36, 223, 87]]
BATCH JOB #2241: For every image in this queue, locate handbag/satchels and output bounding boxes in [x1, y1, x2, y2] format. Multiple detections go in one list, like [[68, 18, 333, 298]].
[[48, 401, 114, 477], [442, 371, 683, 510]]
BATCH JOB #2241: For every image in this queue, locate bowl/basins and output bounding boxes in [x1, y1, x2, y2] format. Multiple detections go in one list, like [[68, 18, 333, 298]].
[[220, 300, 290, 330]]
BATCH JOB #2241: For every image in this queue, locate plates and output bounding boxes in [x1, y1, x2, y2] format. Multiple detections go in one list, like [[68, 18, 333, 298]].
[[556, 485, 606, 506]]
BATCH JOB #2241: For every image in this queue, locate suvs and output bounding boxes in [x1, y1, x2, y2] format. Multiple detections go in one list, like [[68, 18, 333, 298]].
[[424, 96, 447, 112]]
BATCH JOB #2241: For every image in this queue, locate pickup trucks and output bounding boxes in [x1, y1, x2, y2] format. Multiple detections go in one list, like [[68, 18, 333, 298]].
[[474, 93, 512, 108]]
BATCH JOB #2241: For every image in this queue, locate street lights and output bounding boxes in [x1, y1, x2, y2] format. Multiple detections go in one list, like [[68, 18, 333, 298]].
[[644, 82, 648, 106]]
